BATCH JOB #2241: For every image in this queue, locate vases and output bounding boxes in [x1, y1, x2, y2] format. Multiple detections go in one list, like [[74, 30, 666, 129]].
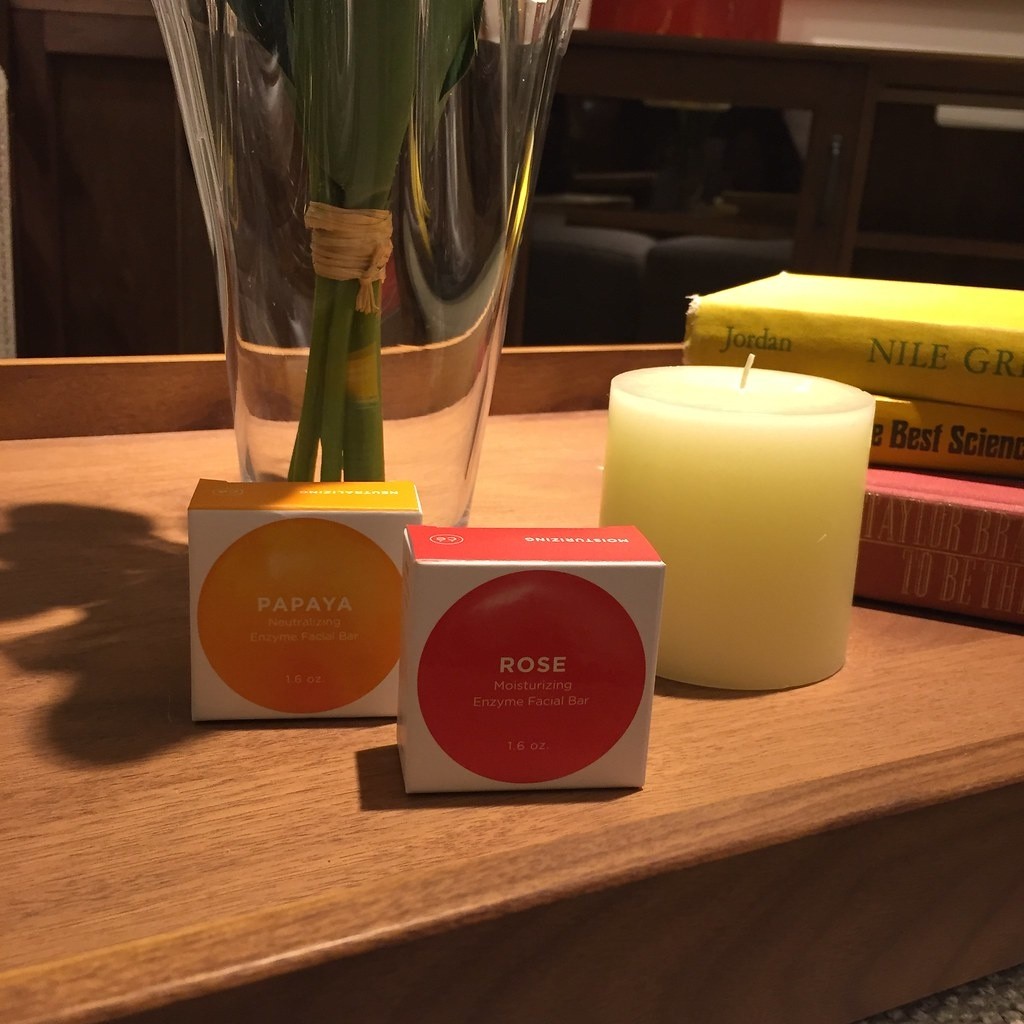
[[151, 1, 587, 526]]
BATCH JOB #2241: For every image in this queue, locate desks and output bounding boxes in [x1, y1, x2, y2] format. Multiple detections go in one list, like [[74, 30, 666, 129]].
[[0, 0, 1024, 366], [0, 341, 1024, 1024]]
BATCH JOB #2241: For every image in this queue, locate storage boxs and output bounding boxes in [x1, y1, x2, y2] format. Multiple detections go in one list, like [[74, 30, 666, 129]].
[[395, 523, 667, 793], [187, 479, 421, 719]]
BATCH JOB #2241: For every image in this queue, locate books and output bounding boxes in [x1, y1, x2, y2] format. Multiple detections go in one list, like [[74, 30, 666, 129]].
[[683, 271, 1024, 624]]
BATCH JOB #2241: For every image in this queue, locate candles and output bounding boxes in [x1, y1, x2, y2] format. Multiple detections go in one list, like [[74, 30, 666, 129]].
[[598, 354, 875, 689]]
